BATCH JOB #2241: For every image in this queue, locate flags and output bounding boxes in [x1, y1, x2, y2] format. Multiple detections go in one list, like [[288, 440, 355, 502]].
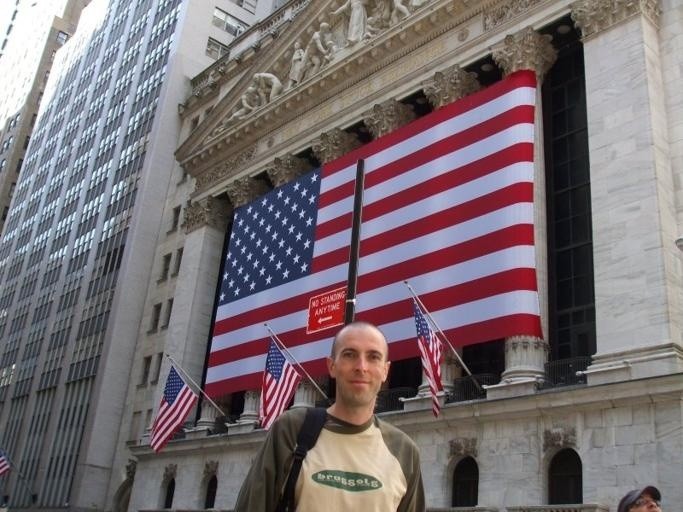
[[0, 449, 10, 477], [205, 69, 543, 397], [150, 366, 198, 453], [413, 297, 442, 419]]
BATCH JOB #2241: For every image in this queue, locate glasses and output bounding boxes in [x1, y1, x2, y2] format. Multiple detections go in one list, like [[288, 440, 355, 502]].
[[630, 498, 659, 507]]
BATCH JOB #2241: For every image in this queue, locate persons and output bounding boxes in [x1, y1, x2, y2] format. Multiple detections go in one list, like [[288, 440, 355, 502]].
[[226, 0, 426, 124], [235, 321, 426, 511]]
